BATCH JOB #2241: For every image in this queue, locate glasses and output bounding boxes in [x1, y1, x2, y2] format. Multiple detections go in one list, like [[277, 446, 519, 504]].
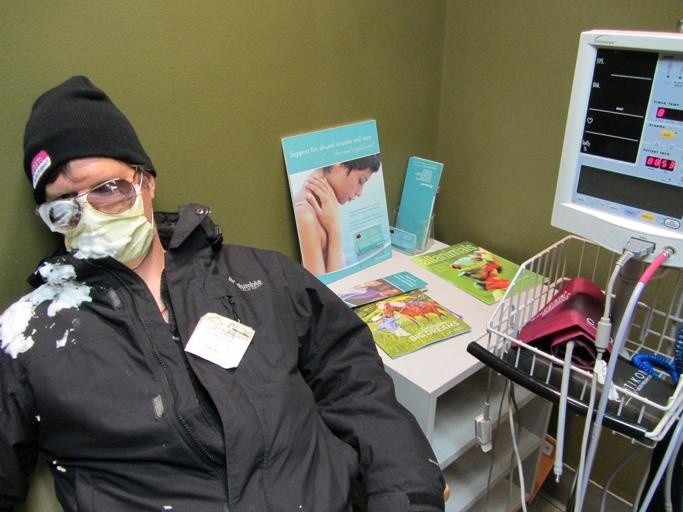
[[35, 166, 138, 233]]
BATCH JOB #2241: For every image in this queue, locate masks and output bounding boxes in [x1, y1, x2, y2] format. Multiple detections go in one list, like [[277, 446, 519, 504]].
[[65, 172, 155, 271]]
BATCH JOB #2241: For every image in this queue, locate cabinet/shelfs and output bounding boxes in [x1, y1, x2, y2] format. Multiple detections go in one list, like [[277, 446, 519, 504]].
[[318, 237, 563, 512]]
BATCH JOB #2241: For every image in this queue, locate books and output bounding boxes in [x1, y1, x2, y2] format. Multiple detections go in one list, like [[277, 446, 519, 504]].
[[392, 152, 445, 256], [409, 239, 548, 310], [339, 286, 473, 364], [275, 116, 393, 284]]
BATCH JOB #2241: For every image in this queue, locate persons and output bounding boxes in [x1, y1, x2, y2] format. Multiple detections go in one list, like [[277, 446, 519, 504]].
[[290, 156, 385, 277], [341, 279, 399, 307], [0, 73, 446, 510]]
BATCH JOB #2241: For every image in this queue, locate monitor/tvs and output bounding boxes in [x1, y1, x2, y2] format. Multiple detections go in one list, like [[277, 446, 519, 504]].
[[550, 28, 683, 269]]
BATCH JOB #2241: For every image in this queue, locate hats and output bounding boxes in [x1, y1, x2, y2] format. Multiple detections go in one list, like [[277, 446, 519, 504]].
[[23, 76, 156, 204]]
